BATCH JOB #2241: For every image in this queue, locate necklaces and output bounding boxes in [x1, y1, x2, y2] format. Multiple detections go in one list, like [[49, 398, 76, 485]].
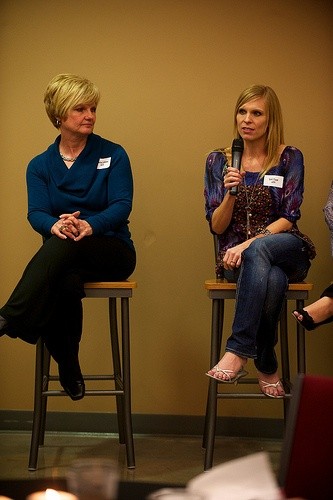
[[242, 170, 260, 229], [60, 153, 77, 162]]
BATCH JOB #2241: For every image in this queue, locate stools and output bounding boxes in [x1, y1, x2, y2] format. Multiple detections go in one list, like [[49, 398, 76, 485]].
[[202, 235, 313, 472], [28, 280, 136, 473]]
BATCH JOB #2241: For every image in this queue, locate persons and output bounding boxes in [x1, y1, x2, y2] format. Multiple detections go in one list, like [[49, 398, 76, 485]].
[[203, 85, 316, 399], [291, 186, 333, 331], [0, 74, 136, 401]]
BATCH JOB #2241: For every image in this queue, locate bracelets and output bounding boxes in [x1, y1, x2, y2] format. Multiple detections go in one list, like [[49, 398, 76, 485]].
[[261, 229, 271, 236]]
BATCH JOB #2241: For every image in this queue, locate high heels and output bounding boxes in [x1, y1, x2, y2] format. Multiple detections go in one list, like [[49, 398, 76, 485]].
[[204, 364, 249, 387], [258, 379, 285, 398], [291, 308, 333, 332]]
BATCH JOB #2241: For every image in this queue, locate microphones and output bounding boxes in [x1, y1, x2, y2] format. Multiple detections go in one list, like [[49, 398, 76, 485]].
[[229, 138, 244, 195]]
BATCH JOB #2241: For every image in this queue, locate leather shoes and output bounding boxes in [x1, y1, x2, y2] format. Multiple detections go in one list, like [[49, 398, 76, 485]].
[[0, 316, 17, 338], [58, 357, 85, 401]]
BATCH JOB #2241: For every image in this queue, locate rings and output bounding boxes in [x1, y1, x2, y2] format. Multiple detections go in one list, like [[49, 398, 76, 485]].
[[60, 225, 64, 230], [231, 261, 236, 263]]
